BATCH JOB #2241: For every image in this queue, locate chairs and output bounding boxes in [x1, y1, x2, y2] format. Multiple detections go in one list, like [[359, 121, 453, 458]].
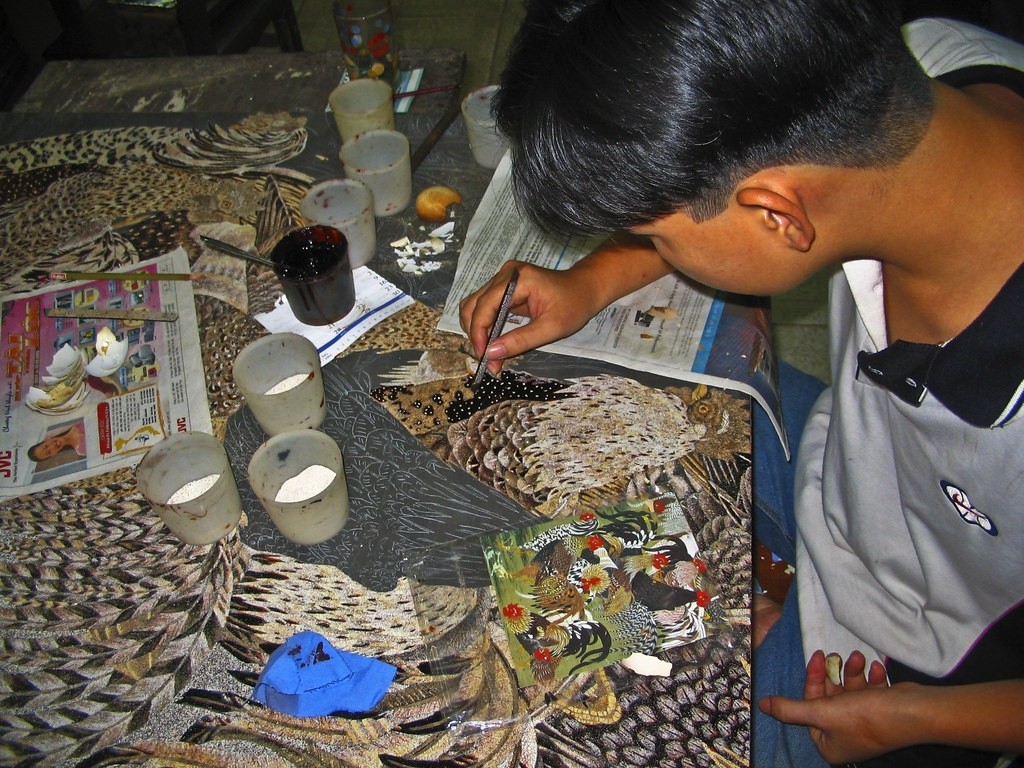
[[48, 0, 304, 58]]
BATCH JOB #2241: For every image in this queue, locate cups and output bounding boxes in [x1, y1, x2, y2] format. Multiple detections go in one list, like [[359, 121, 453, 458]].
[[232, 332, 350, 547], [330, 0, 401, 91], [137, 427, 243, 545], [458, 84, 513, 170], [267, 77, 414, 327]]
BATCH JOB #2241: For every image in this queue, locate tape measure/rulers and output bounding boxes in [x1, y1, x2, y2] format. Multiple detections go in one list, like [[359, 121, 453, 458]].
[[44, 307, 179, 322]]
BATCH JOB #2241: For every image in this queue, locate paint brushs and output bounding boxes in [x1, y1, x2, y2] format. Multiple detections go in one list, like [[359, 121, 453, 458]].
[[198, 234, 297, 273], [473, 269, 522, 386], [50, 273, 204, 280]]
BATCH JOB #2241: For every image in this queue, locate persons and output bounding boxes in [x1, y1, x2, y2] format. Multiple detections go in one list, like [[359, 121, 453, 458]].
[[457, 0, 1024, 768]]
